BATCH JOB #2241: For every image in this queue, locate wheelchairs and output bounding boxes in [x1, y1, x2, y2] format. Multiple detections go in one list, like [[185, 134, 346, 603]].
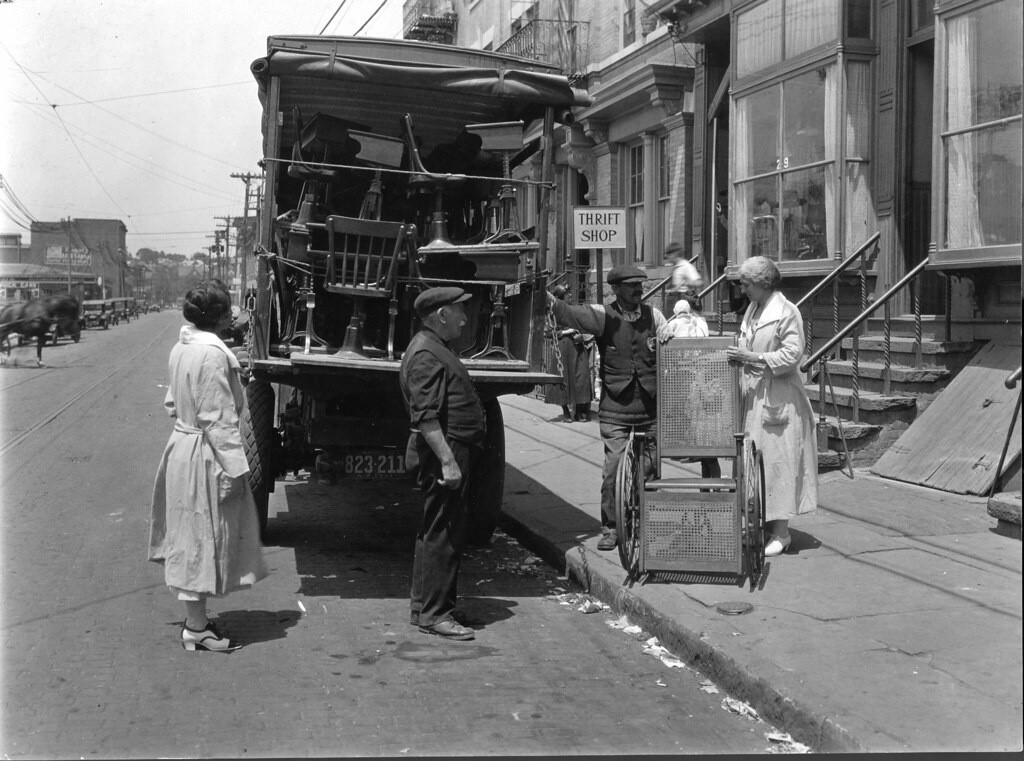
[[615, 335, 765, 585]]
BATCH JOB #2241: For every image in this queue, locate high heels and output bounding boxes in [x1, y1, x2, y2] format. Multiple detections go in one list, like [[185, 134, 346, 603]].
[[181, 619, 242, 652], [764, 533, 791, 557]]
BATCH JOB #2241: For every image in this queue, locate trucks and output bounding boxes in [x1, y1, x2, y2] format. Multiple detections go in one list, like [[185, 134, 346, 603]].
[[241, 34, 576, 549]]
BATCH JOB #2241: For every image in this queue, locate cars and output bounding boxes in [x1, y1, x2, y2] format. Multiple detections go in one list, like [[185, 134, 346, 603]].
[[80, 300, 108, 330], [213, 305, 243, 346], [105, 301, 118, 326], [110, 297, 130, 323], [17, 313, 80, 346], [127, 297, 170, 320]]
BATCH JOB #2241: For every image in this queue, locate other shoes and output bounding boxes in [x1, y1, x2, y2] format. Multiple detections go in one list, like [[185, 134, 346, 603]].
[[743, 531, 769, 546], [597, 527, 618, 551], [563, 417, 573, 423], [573, 415, 587, 422]]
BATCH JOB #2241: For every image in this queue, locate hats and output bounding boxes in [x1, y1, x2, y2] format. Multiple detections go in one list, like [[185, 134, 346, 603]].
[[553, 284, 573, 303], [607, 265, 648, 283], [414, 285, 472, 318]]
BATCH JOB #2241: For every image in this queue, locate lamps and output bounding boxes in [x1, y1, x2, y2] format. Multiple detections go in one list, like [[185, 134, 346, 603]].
[[670, 0, 707, 20]]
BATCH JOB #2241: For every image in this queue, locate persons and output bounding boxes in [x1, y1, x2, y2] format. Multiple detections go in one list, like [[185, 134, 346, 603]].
[[400, 287, 487, 640], [545, 265, 675, 550], [666, 242, 704, 312], [544, 285, 592, 422], [727, 256, 818, 555], [668, 300, 710, 337], [148, 279, 271, 652]]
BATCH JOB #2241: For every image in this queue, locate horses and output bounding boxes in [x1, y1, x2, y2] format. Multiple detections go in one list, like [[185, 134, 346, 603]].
[[0, 293, 81, 367]]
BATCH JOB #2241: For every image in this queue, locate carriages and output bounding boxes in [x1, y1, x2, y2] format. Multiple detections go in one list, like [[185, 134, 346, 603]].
[[0, 295, 79, 368]]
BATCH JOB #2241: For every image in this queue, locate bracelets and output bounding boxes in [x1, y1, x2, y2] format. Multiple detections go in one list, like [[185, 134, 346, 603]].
[[759, 353, 763, 362]]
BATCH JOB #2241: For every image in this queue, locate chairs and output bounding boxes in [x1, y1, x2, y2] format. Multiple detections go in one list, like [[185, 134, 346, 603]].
[[284, 104, 340, 227], [321, 213, 411, 363], [402, 111, 470, 251]]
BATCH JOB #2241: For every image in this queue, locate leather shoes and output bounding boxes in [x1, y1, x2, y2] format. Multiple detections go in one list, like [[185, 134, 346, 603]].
[[407, 610, 472, 628], [420, 614, 476, 640]]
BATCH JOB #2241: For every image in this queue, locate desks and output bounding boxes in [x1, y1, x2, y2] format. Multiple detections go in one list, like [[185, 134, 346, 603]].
[[463, 119, 534, 246], [458, 251, 527, 363], [298, 112, 407, 222], [302, 246, 407, 363]]
